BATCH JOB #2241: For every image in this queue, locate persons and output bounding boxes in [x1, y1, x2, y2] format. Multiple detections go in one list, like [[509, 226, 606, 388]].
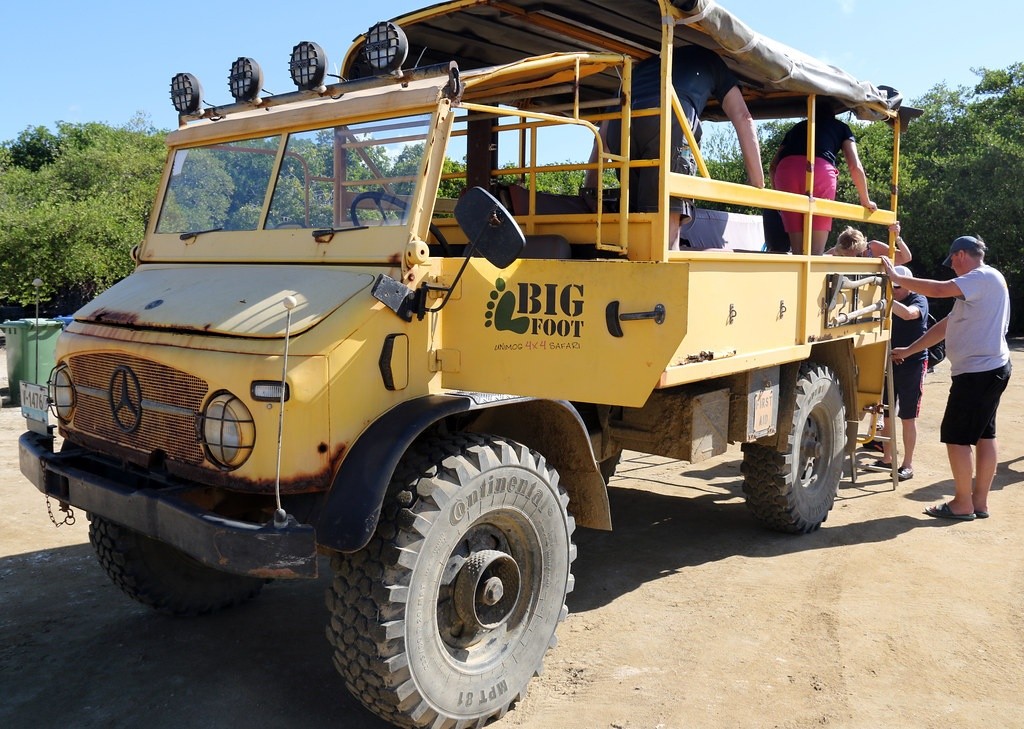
[[585, 44, 765, 251], [824, 221, 912, 266], [878, 236, 1013, 521], [769, 96, 877, 256], [863, 266, 928, 480]]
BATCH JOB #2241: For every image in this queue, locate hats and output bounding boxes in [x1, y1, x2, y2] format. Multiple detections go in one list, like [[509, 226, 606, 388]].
[[942, 236, 988, 267], [893, 265, 913, 286]]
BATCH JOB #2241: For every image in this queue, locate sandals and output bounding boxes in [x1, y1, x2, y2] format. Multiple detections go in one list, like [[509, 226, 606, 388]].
[[864, 440, 884, 452]]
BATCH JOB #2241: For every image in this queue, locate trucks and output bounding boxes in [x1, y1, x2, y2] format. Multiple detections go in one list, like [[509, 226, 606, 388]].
[[18, 0, 902, 729]]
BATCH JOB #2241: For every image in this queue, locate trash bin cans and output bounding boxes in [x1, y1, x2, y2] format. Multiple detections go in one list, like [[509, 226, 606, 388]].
[[0, 315, 74, 407]]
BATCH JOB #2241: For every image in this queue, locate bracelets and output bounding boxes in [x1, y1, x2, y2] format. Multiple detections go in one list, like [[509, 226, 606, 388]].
[[896, 237, 902, 244]]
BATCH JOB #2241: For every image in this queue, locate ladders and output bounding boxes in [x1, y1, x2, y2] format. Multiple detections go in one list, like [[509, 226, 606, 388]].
[[851, 273, 899, 491]]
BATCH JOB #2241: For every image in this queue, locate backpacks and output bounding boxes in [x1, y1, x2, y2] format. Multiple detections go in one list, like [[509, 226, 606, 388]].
[[927, 314, 946, 368]]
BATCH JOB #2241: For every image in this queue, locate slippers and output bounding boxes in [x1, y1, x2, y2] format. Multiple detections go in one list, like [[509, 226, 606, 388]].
[[975, 511, 989, 517], [925, 502, 976, 520]]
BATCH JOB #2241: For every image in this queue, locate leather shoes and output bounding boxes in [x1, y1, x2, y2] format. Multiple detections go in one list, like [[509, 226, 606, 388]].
[[863, 460, 893, 472], [890, 466, 913, 480]]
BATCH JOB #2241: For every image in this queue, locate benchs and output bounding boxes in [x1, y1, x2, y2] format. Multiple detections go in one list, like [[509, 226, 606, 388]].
[[462, 235, 572, 259]]
